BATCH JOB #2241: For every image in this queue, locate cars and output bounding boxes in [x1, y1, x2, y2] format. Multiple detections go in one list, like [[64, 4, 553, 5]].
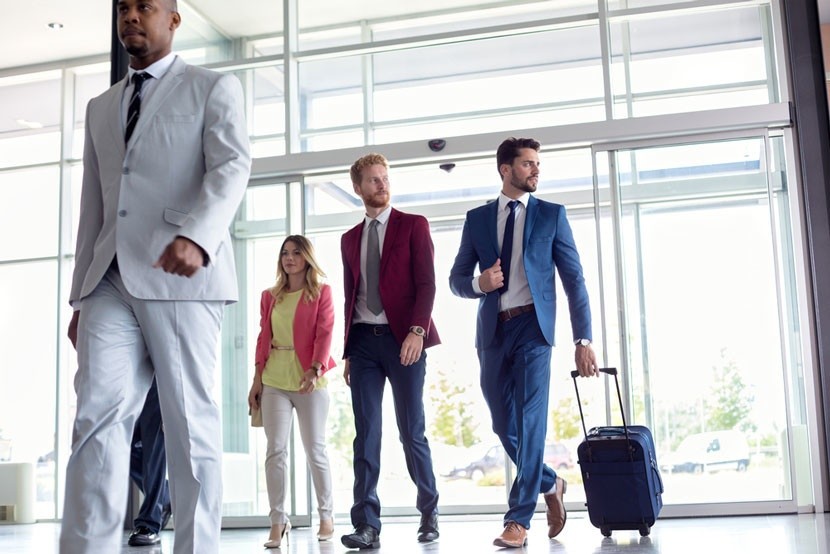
[[543, 444, 573, 470], [661, 431, 753, 474], [466, 444, 502, 480]]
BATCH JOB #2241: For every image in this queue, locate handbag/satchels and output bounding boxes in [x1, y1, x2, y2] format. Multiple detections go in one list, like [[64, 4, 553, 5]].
[[249, 383, 264, 427]]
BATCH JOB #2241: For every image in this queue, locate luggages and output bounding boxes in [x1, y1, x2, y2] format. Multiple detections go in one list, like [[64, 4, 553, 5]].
[[570, 368, 663, 538]]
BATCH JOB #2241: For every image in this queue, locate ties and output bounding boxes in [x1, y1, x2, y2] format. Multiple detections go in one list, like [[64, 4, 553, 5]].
[[366, 220, 384, 316], [498, 200, 521, 294], [125, 72, 154, 149]]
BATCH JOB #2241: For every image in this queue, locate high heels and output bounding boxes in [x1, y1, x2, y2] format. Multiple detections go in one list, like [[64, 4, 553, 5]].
[[264, 521, 292, 547], [317, 516, 334, 540]]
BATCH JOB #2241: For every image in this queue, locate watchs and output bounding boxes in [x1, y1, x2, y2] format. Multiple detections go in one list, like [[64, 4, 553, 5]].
[[575, 339, 591, 346], [308, 366, 322, 378]]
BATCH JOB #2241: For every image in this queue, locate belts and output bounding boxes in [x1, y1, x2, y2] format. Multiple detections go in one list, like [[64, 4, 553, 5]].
[[498, 303, 534, 323]]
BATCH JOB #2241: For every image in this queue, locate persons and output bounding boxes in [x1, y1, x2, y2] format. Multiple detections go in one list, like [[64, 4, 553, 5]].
[[409, 326, 427, 338], [341, 154, 440, 550], [248, 236, 337, 548], [449, 138, 600, 548], [59, 0, 252, 554], [129, 383, 176, 548]]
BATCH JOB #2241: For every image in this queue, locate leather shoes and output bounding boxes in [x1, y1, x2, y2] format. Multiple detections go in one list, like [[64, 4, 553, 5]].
[[351, 324, 391, 336], [544, 476, 567, 539], [128, 526, 161, 546], [341, 523, 380, 549], [161, 502, 172, 530], [417, 506, 440, 541], [493, 522, 528, 548]]
